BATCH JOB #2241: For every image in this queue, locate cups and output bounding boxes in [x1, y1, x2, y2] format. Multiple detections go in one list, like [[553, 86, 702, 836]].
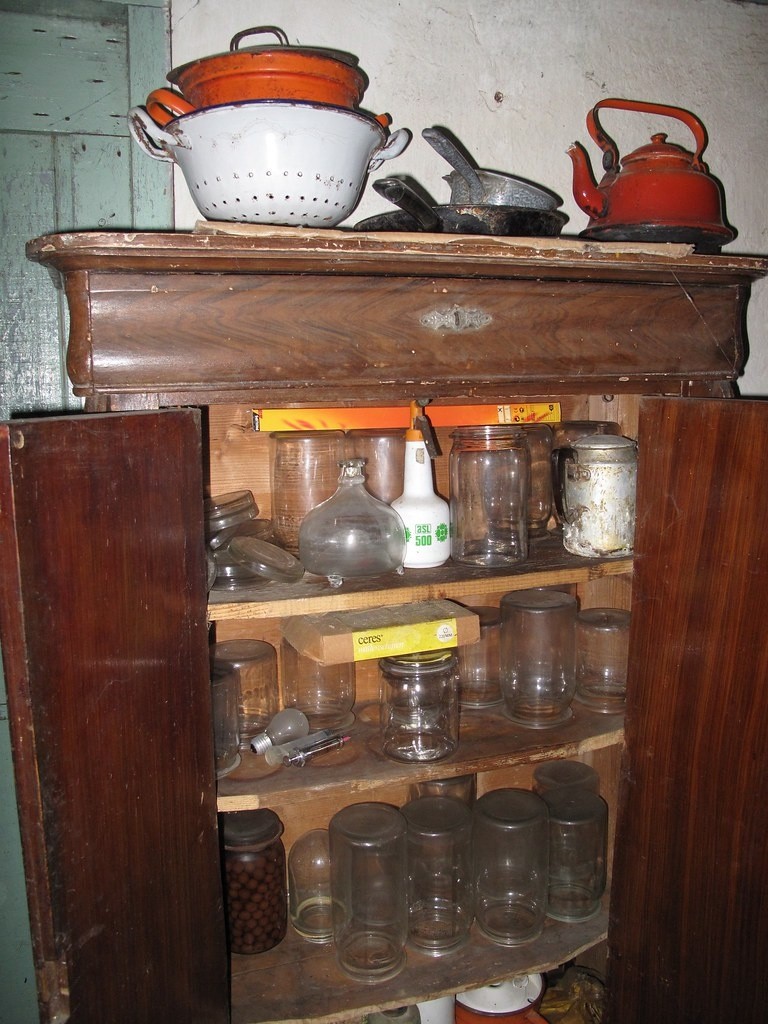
[[455, 973, 549, 1024]]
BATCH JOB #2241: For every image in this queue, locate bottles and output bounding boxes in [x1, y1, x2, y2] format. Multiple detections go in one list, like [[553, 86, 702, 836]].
[[527, 423, 554, 540], [280, 638, 356, 734], [378, 648, 458, 764], [500, 590, 577, 729], [554, 419, 622, 534], [328, 802, 407, 985], [448, 426, 531, 567], [532, 760, 600, 804], [472, 788, 548, 949], [209, 663, 243, 781], [269, 430, 407, 557], [454, 606, 503, 709], [214, 639, 279, 751], [540, 791, 608, 924], [577, 609, 630, 714], [406, 773, 477, 811], [400, 795, 477, 953]]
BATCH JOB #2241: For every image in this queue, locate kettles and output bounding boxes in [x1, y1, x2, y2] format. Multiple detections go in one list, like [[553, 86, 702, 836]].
[[566, 98, 734, 255]]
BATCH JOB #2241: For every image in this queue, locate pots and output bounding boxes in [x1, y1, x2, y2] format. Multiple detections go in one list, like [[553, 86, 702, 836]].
[[128, 27, 570, 238]]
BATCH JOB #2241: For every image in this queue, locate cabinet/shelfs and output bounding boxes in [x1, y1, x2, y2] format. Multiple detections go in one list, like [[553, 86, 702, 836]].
[[0, 225, 768, 1023]]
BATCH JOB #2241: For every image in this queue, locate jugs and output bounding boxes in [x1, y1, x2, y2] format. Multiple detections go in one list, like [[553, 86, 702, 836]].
[[551, 423, 637, 558]]
